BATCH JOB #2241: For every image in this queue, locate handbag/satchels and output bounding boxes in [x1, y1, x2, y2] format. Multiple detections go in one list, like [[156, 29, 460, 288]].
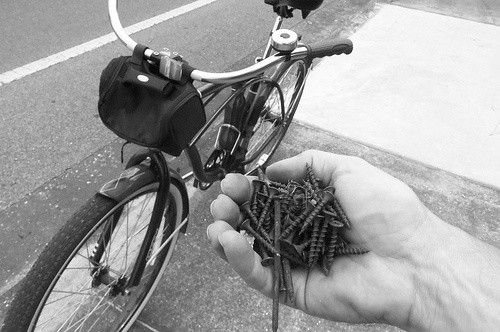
[[98, 43, 206, 158]]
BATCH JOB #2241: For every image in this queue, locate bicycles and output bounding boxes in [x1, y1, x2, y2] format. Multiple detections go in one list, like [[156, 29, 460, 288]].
[[0, 1, 353, 331]]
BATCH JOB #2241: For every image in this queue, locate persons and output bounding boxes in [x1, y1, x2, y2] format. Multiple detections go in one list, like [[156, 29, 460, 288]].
[[205, 148, 500, 332]]
